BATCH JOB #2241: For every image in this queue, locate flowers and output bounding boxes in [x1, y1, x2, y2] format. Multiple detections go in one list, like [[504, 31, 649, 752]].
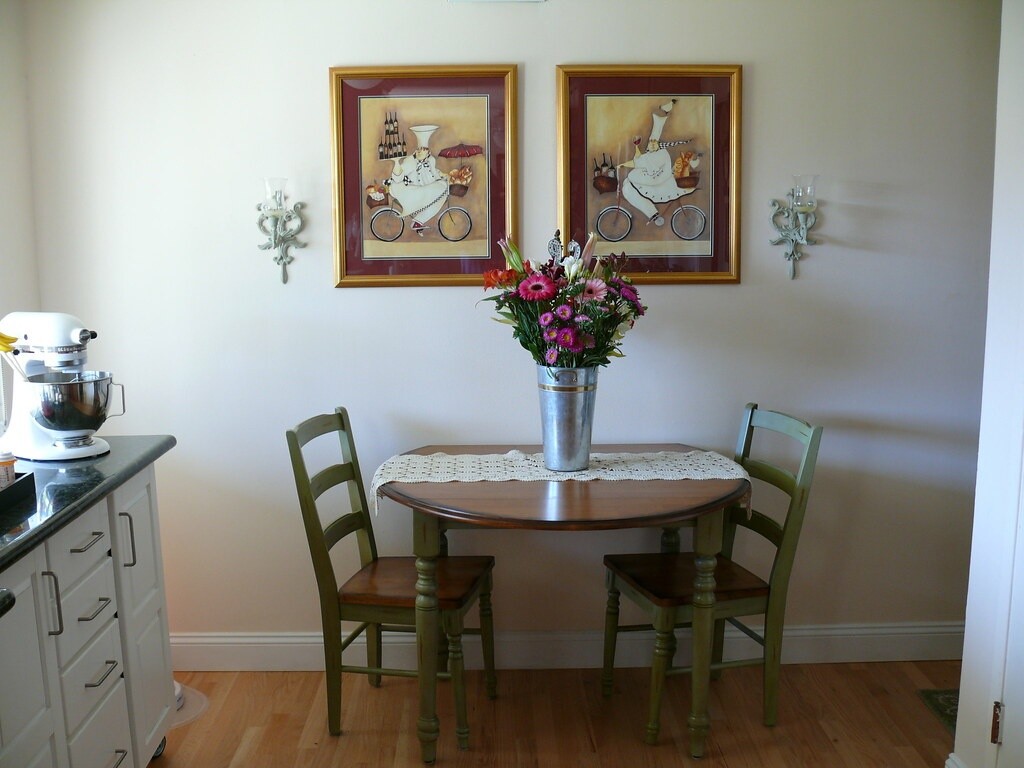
[[480, 228, 646, 371]]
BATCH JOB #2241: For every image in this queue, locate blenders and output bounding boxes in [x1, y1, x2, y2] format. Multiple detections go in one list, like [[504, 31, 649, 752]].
[[0, 312, 126, 461]]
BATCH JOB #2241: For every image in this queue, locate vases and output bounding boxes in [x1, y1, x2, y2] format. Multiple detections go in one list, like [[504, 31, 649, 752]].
[[538, 361, 599, 472]]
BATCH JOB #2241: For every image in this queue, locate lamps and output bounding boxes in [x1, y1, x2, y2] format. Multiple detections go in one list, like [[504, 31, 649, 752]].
[[254, 177, 310, 283], [767, 172, 821, 278]]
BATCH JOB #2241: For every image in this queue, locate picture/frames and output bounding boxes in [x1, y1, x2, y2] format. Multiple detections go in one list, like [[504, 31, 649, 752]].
[[554, 61, 740, 287], [326, 66, 519, 288]]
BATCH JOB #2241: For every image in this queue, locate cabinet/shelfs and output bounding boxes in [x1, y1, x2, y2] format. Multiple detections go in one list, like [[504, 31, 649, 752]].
[[0, 464, 176, 767]]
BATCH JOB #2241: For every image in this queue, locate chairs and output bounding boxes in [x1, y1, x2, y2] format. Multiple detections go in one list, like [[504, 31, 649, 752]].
[[285, 406, 498, 752], [597, 401, 824, 744]]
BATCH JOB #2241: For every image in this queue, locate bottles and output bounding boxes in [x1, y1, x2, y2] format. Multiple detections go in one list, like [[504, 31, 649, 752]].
[[0, 452, 18, 487]]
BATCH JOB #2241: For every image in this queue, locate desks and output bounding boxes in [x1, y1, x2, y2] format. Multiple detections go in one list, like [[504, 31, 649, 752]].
[[372, 443, 752, 763]]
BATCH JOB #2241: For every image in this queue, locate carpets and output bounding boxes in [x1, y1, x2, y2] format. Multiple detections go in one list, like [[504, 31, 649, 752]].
[[916, 685, 961, 739]]
